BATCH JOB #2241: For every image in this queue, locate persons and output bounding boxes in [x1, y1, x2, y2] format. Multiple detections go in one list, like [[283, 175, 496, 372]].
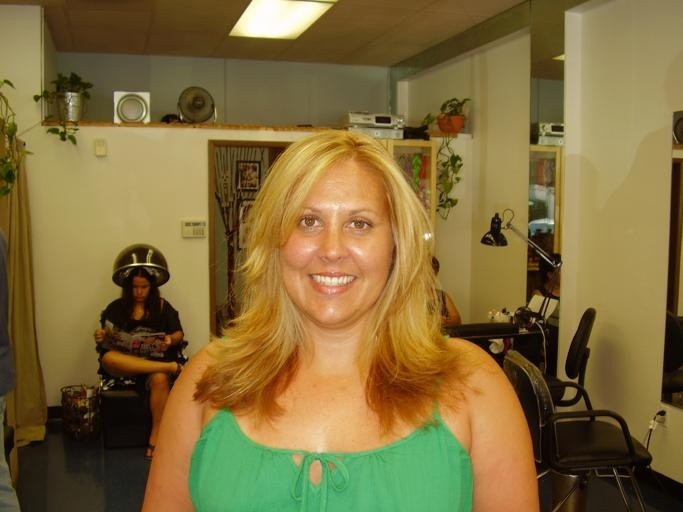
[[140, 130, 541, 511], [426, 256, 461, 327], [533, 252, 560, 334], [92, 266, 185, 461]]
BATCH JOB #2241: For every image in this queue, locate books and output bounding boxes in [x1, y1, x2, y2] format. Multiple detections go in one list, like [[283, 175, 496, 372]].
[[100, 320, 165, 358]]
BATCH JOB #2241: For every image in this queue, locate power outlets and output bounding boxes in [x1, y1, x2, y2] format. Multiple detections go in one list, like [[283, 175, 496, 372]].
[[655, 404, 669, 429]]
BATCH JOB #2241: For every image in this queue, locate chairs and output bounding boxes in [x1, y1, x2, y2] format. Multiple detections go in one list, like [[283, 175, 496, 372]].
[[95, 339, 189, 454], [526, 295, 558, 324], [503, 308, 652, 512]]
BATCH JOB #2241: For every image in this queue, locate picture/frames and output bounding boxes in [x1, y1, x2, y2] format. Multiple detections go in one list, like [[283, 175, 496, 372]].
[[236, 161, 262, 251]]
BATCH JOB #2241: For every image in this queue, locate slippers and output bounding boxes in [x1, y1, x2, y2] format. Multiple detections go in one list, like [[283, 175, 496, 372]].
[[144, 441, 155, 461]]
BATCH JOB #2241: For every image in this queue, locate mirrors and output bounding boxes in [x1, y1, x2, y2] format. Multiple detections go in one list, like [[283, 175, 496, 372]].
[[208, 138, 295, 337], [528, 142, 560, 271]]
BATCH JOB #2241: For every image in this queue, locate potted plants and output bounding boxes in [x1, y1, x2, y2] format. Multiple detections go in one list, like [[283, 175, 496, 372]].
[[433, 97, 471, 213], [34, 72, 94, 145]]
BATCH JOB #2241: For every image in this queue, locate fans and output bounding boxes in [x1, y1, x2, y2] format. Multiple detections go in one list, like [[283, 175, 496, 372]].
[[176, 86, 216, 123]]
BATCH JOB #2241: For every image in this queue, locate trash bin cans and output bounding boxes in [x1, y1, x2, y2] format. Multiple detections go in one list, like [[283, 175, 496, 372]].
[[60, 385, 99, 447]]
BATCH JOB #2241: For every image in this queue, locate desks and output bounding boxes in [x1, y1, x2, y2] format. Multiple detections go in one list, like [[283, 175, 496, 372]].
[[437, 322, 558, 406]]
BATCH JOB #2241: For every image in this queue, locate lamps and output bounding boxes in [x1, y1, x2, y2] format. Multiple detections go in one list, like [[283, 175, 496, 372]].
[[479, 213, 562, 329]]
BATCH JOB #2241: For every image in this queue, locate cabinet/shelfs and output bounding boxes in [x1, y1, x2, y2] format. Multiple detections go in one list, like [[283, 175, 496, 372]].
[[380, 139, 437, 230]]
